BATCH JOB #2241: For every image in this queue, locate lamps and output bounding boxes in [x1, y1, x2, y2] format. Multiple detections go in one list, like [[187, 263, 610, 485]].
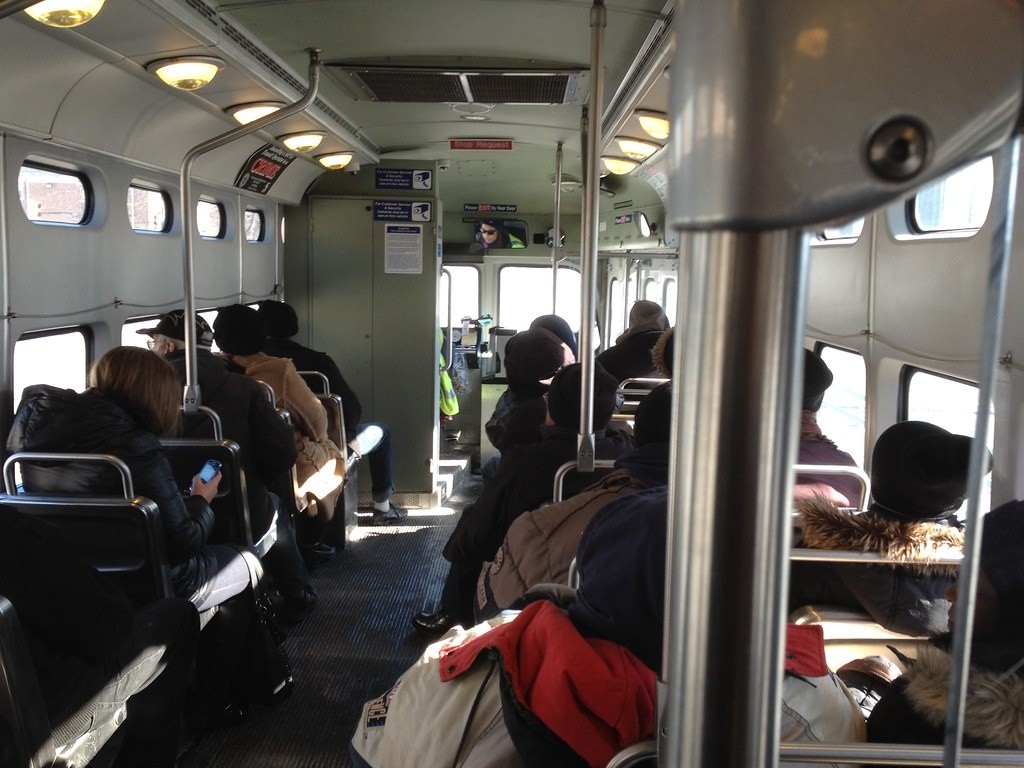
[[600, 157, 640, 175], [614, 137, 661, 159], [24, 0, 106, 28], [227, 103, 287, 126], [277, 131, 327, 153], [314, 151, 356, 170], [636, 109, 671, 139], [147, 57, 226, 91]]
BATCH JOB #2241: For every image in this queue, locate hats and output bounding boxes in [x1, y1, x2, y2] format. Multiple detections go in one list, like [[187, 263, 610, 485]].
[[548, 363, 620, 429], [577, 486, 667, 643], [505, 326, 573, 398], [259, 300, 298, 337], [962, 495, 1024, 645], [214, 304, 268, 355], [137, 308, 213, 347], [872, 420, 993, 522], [615, 300, 671, 345], [530, 314, 578, 358], [799, 345, 834, 415]]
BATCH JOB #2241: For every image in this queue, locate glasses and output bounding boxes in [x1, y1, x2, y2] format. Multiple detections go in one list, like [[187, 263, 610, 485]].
[[480, 229, 497, 235]]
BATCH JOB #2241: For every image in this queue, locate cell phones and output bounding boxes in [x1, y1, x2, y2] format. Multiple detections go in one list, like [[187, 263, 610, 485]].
[[190, 460, 222, 491]]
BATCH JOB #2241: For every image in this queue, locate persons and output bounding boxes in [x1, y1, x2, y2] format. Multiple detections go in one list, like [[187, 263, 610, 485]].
[[3, 300, 1022, 768], [474, 219, 524, 249]]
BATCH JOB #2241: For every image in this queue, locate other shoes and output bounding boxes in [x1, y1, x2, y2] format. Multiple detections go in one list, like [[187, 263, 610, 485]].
[[191, 702, 249, 729], [302, 541, 335, 558]]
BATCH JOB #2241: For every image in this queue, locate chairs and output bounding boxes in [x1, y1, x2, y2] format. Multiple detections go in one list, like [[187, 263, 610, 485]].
[[549, 365, 1024, 768], [0, 371, 363, 768]]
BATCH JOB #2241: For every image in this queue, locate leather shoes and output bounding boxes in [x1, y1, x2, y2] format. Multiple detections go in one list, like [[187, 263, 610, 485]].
[[282, 584, 317, 623], [373, 502, 408, 524], [413, 610, 475, 637]]
